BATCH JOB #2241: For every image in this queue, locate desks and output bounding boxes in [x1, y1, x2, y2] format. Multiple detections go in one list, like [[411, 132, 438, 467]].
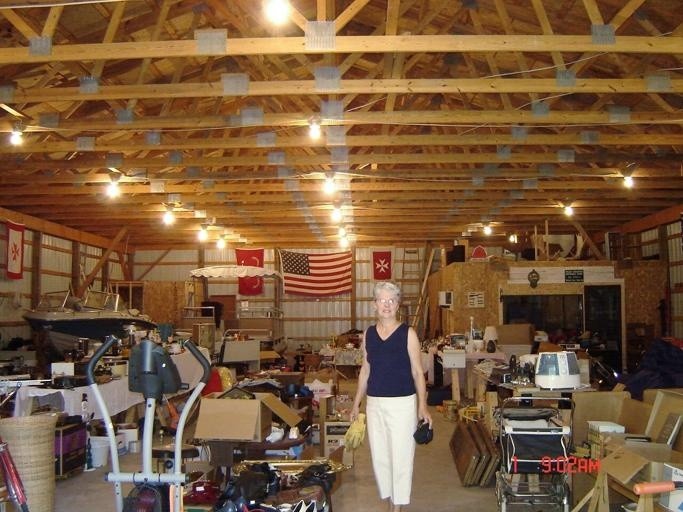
[[569, 447, 682, 512], [17, 346, 211, 425], [333, 346, 364, 380], [472, 367, 598, 408]]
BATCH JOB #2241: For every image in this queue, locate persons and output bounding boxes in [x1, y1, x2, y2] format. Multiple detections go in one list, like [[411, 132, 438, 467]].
[[348, 281, 434, 512]]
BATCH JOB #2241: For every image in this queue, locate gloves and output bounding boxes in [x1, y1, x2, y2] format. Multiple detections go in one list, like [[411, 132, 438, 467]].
[[344, 413, 366, 452]]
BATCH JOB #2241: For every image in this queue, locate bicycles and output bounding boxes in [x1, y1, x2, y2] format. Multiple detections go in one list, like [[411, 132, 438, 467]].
[[0, 382, 29, 512]]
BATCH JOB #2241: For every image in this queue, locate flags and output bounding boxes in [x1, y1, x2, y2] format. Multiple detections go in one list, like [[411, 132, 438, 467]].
[[4, 217, 26, 281], [373, 251, 392, 280], [235, 247, 265, 296], [277, 249, 354, 298]]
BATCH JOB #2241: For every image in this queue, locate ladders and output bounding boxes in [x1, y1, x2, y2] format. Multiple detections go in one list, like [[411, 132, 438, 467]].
[[399, 242, 426, 343]]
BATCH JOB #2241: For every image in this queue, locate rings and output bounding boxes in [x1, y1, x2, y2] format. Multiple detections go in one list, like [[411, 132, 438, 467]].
[[424, 417, 428, 419]]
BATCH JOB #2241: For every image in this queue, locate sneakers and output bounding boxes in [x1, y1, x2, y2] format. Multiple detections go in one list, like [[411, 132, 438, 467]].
[[266, 420, 311, 443], [288, 383, 313, 397]]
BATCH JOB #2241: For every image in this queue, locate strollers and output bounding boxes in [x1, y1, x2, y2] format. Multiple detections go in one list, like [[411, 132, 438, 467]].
[[494, 396, 575, 512]]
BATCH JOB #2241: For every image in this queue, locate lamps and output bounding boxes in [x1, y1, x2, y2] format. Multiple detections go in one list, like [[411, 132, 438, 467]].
[[482, 163, 639, 246], [307, 113, 353, 251], [7, 120, 229, 252]]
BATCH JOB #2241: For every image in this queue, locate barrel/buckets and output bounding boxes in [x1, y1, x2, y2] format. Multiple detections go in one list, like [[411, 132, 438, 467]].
[[92, 438, 111, 467]]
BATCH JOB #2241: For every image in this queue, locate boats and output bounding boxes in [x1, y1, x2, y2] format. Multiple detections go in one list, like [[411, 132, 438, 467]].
[[25, 289, 160, 368]]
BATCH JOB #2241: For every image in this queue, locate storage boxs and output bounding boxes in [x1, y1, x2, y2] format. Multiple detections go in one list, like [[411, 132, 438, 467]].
[[117, 424, 140, 453], [54, 421, 86, 479], [222, 340, 262, 376], [193, 391, 303, 442], [324, 420, 355, 468]]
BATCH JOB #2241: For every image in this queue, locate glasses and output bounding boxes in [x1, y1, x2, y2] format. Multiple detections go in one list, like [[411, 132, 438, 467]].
[[378, 299, 394, 304]]
